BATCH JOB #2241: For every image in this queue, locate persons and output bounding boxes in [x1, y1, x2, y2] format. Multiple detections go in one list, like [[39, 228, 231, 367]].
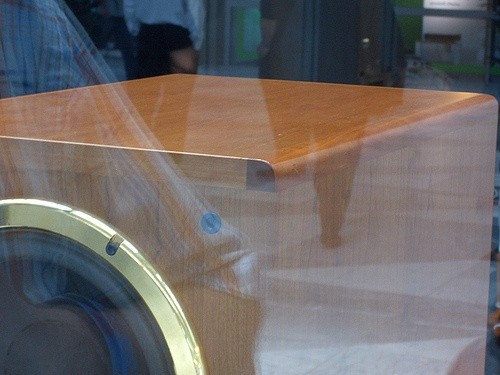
[[105, 1, 207, 167], [254, 1, 399, 251]]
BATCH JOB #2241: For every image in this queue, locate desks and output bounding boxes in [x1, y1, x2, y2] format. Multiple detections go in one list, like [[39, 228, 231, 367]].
[[0, 75, 498, 375]]
